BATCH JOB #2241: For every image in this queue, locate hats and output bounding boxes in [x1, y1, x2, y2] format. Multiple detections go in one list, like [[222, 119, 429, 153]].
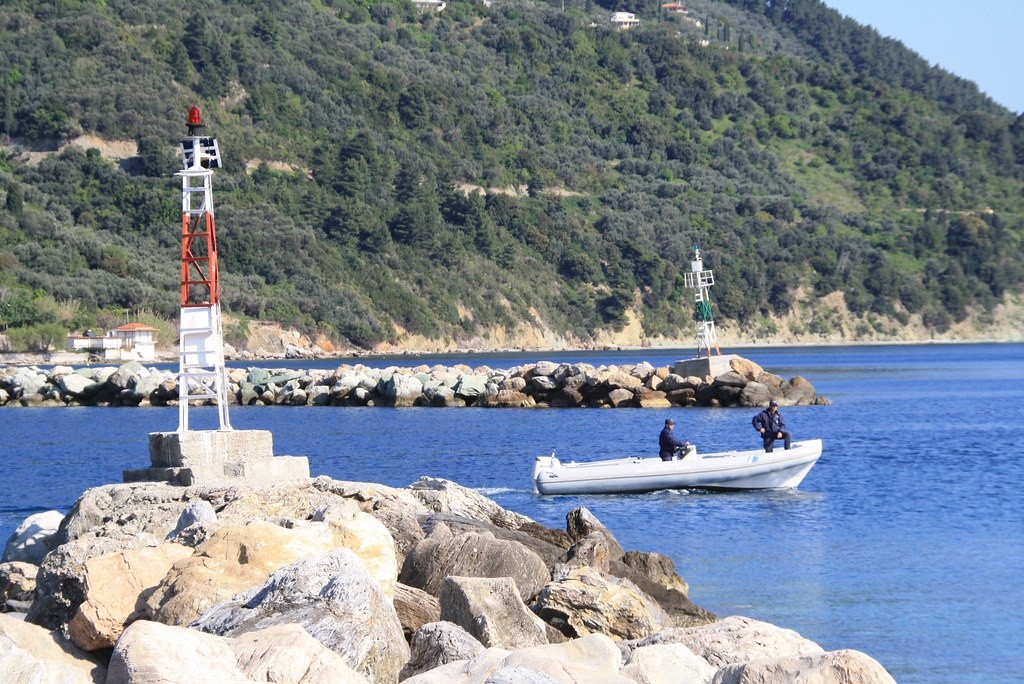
[[770, 400, 777, 406], [665, 418, 675, 425]]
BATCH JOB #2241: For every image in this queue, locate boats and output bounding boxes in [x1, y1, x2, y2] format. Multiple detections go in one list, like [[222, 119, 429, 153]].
[[533, 435, 823, 496]]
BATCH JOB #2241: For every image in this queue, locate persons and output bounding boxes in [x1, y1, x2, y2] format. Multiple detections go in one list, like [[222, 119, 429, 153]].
[[752, 399, 792, 453], [659, 419, 689, 461]]
